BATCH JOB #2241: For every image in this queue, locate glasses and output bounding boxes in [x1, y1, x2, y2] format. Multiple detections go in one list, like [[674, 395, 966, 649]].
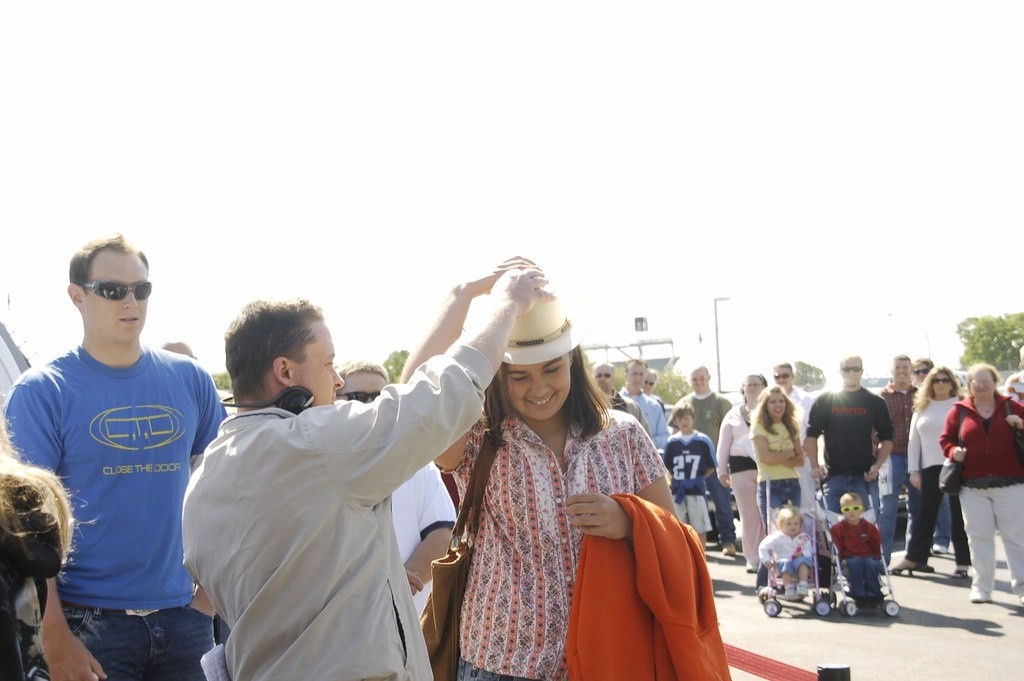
[[914, 368, 930, 375], [841, 505, 863, 513], [841, 367, 863, 373], [775, 373, 791, 379], [643, 380, 654, 387], [595, 373, 612, 378], [932, 377, 952, 384], [335, 391, 382, 404], [74, 276, 153, 301]]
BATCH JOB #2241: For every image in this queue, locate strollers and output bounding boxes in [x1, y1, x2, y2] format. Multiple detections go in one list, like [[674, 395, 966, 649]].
[[819, 468, 901, 620], [756, 465, 833, 619]]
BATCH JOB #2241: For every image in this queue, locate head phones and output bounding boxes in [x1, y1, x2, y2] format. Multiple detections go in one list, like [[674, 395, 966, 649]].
[[219, 385, 315, 418]]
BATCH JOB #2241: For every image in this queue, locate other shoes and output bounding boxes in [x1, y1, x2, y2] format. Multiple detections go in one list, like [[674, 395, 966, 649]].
[[917, 561, 934, 571], [856, 596, 879, 608], [723, 542, 734, 556], [786, 588, 797, 599], [969, 588, 994, 604], [797, 583, 808, 597], [746, 562, 757, 572]]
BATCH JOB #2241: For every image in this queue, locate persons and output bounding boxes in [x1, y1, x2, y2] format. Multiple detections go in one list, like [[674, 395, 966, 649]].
[[182, 268, 556, 681], [663, 403, 719, 552], [402, 256, 679, 681], [333, 360, 458, 620], [758, 506, 814, 599], [666, 366, 737, 556], [0, 236, 226, 681], [618, 359, 670, 451], [878, 345, 1024, 607], [805, 354, 895, 566], [717, 362, 819, 596], [162, 341, 198, 361], [829, 492, 885, 600], [0, 394, 75, 681], [592, 361, 653, 441]]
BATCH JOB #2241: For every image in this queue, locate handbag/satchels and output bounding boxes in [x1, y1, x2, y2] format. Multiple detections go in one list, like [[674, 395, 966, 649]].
[[938, 403, 965, 494], [420, 428, 500, 681], [1004, 399, 1024, 458]]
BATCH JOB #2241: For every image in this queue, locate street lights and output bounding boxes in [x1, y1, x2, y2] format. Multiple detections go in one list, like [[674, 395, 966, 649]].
[[713, 295, 732, 394], [887, 312, 931, 360]]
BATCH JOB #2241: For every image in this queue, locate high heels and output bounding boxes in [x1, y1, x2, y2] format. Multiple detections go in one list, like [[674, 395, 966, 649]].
[[954, 566, 967, 578], [887, 561, 916, 576]]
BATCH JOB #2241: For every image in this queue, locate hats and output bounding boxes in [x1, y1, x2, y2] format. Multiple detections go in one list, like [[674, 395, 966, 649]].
[[498, 289, 581, 367]]
